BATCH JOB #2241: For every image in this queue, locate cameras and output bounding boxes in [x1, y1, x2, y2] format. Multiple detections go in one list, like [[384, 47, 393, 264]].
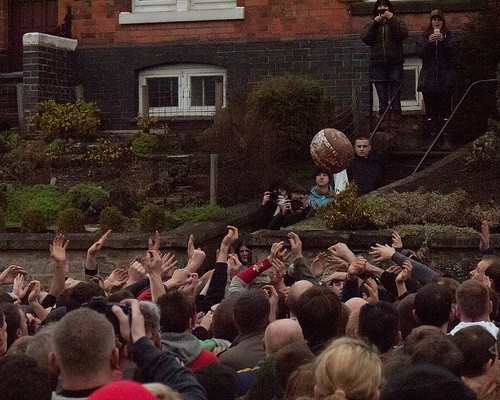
[[266, 189, 281, 202], [81, 296, 128, 334], [376, 9, 387, 14], [287, 197, 303, 210]]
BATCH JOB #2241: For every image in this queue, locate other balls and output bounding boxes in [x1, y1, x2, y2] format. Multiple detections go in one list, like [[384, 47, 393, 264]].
[[310, 129, 354, 174]]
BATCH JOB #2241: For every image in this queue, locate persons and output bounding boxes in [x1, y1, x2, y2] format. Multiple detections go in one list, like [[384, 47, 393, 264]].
[[0, 221, 499, 399], [346, 135, 383, 199], [257, 181, 290, 230], [415, 9, 465, 152], [272, 186, 317, 228], [304, 167, 338, 209], [359, 0, 402, 113]]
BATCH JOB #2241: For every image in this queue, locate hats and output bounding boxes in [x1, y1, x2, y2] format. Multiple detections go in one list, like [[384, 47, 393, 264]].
[[430, 9, 445, 19]]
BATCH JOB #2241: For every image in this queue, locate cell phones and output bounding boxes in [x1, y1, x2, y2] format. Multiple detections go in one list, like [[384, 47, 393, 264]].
[[435, 29, 440, 38]]
